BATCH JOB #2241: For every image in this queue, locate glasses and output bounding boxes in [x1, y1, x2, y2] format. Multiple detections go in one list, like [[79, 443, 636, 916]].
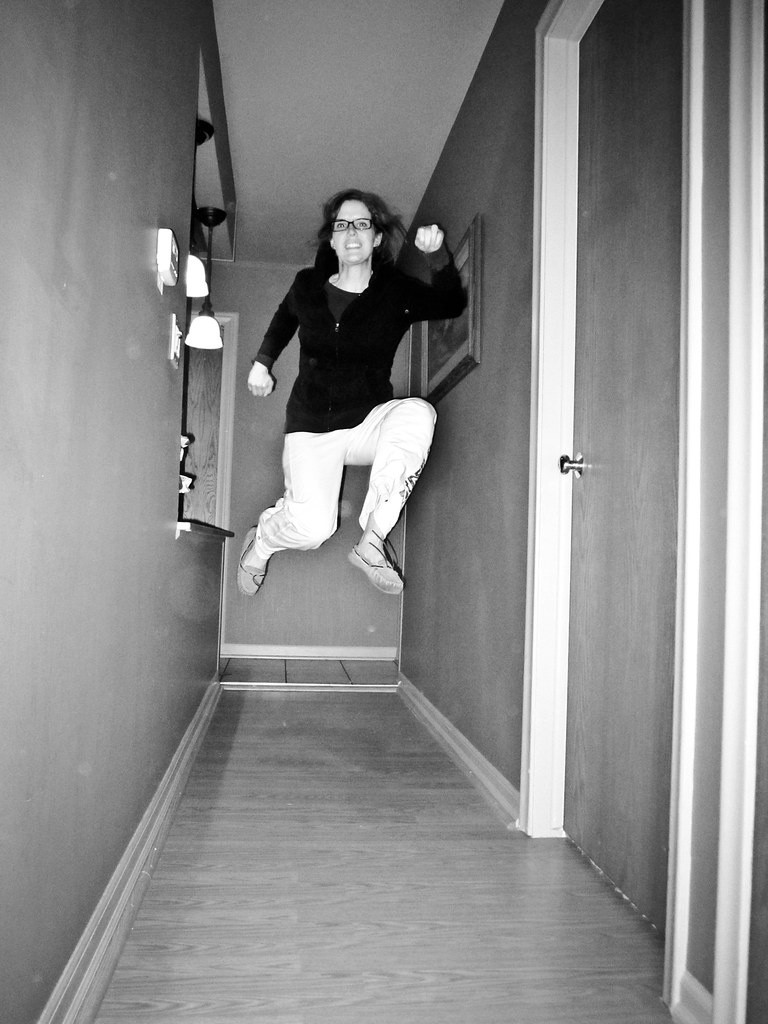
[[330, 218, 372, 232]]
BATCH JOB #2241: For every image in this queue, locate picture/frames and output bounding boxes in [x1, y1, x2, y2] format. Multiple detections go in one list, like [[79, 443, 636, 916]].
[[420, 210, 480, 407]]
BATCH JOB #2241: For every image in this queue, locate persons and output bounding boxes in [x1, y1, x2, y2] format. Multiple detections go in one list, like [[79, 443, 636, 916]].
[[236, 188, 468, 596]]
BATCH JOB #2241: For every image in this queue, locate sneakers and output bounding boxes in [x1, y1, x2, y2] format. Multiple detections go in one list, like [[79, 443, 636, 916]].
[[348, 544, 403, 594], [236, 526, 265, 596]]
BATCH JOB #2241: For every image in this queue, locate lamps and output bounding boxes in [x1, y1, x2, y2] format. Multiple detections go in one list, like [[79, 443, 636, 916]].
[[185, 205, 229, 348], [187, 119, 216, 298]]
